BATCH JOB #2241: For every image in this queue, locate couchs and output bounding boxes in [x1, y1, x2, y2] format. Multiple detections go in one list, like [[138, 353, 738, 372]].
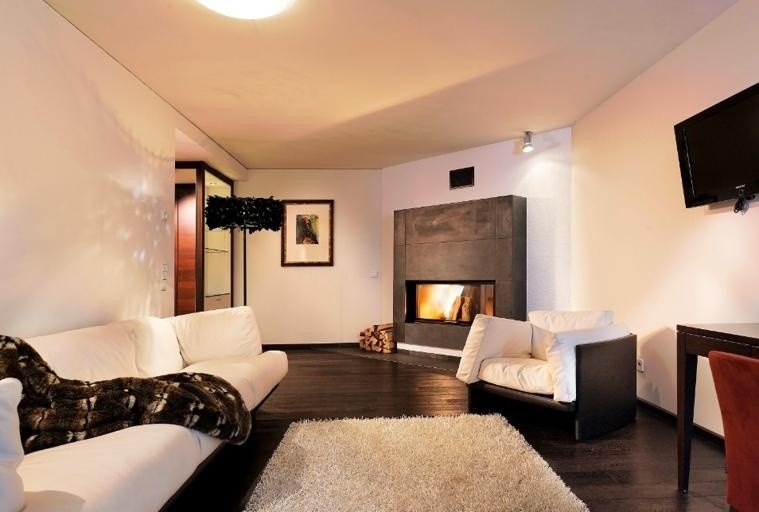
[[0, 306, 289, 512]]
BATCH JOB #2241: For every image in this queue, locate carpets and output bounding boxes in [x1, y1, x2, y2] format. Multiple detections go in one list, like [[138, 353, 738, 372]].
[[234, 412, 590, 512]]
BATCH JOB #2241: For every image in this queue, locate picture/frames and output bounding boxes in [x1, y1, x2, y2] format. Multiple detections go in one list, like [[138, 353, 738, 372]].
[[280, 199, 334, 266]]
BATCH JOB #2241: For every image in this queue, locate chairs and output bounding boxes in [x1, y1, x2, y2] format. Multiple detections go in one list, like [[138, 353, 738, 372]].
[[455, 310, 638, 443], [705, 349, 759, 512]]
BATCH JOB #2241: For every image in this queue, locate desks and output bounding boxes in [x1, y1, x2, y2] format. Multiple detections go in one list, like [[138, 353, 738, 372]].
[[676, 321, 759, 493]]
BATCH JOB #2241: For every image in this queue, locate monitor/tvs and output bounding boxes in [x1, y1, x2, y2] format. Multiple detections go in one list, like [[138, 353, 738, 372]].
[[674, 82, 759, 208]]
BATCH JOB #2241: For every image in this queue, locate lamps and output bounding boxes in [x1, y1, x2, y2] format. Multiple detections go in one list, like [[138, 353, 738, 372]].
[[522, 131, 535, 154], [202, 194, 283, 306]]
